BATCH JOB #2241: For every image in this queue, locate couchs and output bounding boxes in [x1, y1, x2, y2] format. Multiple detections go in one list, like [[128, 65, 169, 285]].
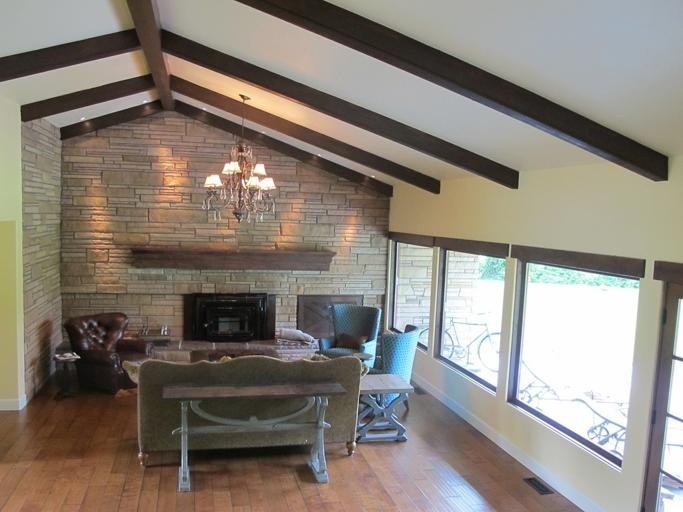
[[136, 356, 362, 467]]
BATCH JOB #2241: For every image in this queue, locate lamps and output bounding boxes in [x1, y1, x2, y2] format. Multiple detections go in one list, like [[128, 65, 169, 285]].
[[201, 95, 277, 224]]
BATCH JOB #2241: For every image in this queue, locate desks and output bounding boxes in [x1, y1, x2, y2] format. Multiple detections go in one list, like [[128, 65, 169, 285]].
[[162, 380, 347, 492], [357, 374, 415, 443], [53, 352, 81, 401]]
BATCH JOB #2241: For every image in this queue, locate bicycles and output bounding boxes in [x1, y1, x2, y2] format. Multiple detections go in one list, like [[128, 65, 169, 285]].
[[417, 309, 502, 372]]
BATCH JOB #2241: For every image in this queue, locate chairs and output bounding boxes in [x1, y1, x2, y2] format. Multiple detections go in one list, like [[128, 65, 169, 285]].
[[318, 303, 421, 415], [64, 313, 158, 394]]
